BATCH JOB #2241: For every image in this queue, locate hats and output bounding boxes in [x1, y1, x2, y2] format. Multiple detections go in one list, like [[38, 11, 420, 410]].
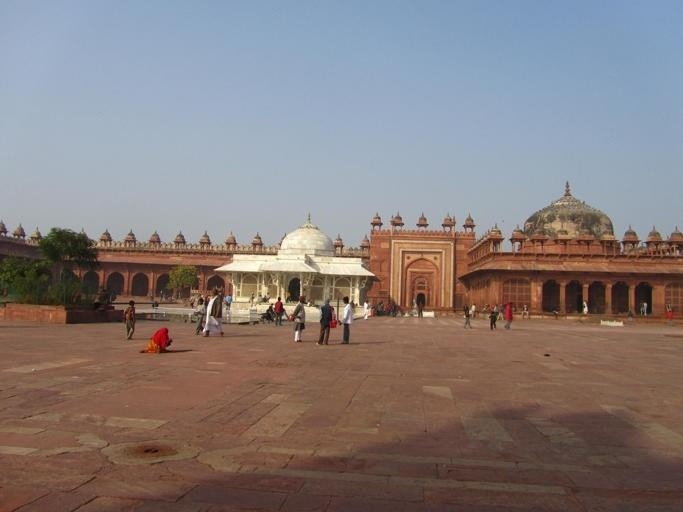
[[299, 296, 305, 301]]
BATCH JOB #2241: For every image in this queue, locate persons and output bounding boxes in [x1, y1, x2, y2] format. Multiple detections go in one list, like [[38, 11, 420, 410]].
[[188, 288, 285, 337], [362, 299, 425, 318], [462, 301, 517, 330], [140, 328, 173, 353], [122, 300, 136, 338], [292, 295, 308, 343], [640, 301, 644, 315], [521, 303, 530, 319], [582, 300, 587, 313], [315, 297, 333, 345], [338, 296, 352, 344], [643, 300, 648, 314]]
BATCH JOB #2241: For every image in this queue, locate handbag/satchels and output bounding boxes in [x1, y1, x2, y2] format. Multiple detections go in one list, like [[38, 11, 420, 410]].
[[331, 320, 337, 328]]
